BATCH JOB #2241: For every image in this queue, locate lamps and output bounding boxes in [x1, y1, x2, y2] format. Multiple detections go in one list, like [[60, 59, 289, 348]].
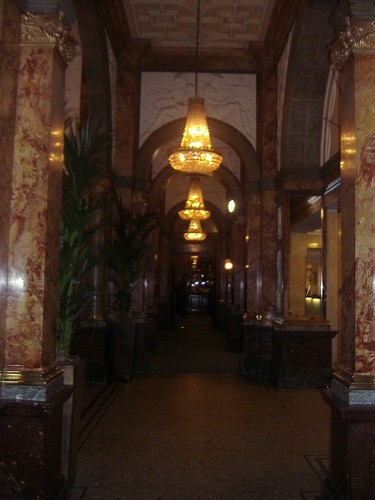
[[184, 218, 207, 241], [167, 0, 223, 177], [177, 171, 212, 222]]
